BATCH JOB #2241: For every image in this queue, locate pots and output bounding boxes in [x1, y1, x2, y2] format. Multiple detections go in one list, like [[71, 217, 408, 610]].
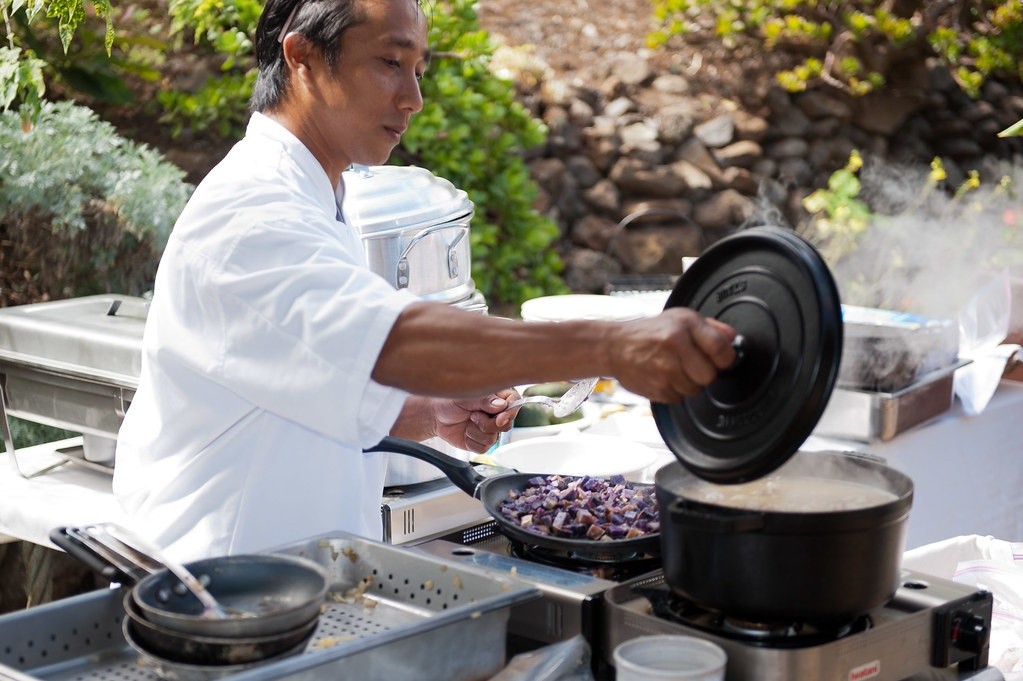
[[656, 449, 913, 619], [339, 164, 475, 306], [51, 520, 329, 681], [362, 434, 661, 559]]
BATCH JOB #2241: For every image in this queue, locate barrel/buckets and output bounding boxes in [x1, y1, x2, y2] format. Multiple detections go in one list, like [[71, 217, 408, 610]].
[[614, 635, 728, 680]]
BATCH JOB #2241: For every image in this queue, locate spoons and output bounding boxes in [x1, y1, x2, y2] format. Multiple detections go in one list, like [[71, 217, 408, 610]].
[[497, 375, 597, 417]]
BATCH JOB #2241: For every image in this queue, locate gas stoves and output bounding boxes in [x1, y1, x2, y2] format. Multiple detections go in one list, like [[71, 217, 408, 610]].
[[366, 461, 993, 681]]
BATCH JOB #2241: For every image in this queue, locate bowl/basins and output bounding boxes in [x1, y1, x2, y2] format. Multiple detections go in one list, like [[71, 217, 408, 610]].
[[832, 304, 958, 394], [520, 289, 673, 321]]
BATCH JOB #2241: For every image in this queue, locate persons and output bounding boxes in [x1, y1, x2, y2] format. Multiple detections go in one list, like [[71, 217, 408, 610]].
[[112, 0, 735, 565]]
